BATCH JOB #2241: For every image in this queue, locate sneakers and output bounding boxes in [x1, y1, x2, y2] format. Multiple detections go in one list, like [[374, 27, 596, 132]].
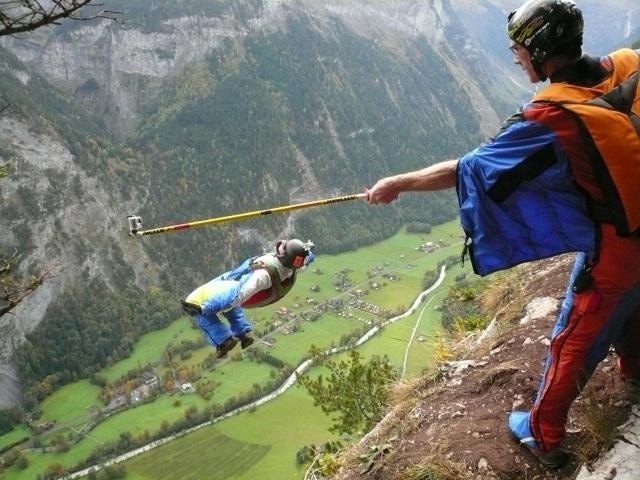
[[239, 330, 253, 349], [214, 335, 238, 358], [509, 411, 564, 470]]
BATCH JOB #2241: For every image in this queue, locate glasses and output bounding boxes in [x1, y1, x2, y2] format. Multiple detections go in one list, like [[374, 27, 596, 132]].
[[508, 43, 520, 56]]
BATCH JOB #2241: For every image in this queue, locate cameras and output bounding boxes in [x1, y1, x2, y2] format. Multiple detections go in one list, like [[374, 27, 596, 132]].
[[128, 216, 142, 231]]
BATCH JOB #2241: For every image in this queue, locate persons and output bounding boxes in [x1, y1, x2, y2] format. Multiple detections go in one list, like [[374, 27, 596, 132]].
[[364, 0, 639, 465], [185, 238, 306, 357]]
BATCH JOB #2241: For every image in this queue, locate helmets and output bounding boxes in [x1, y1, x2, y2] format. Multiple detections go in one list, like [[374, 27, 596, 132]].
[[503, 0, 584, 67], [274, 239, 315, 268]]
